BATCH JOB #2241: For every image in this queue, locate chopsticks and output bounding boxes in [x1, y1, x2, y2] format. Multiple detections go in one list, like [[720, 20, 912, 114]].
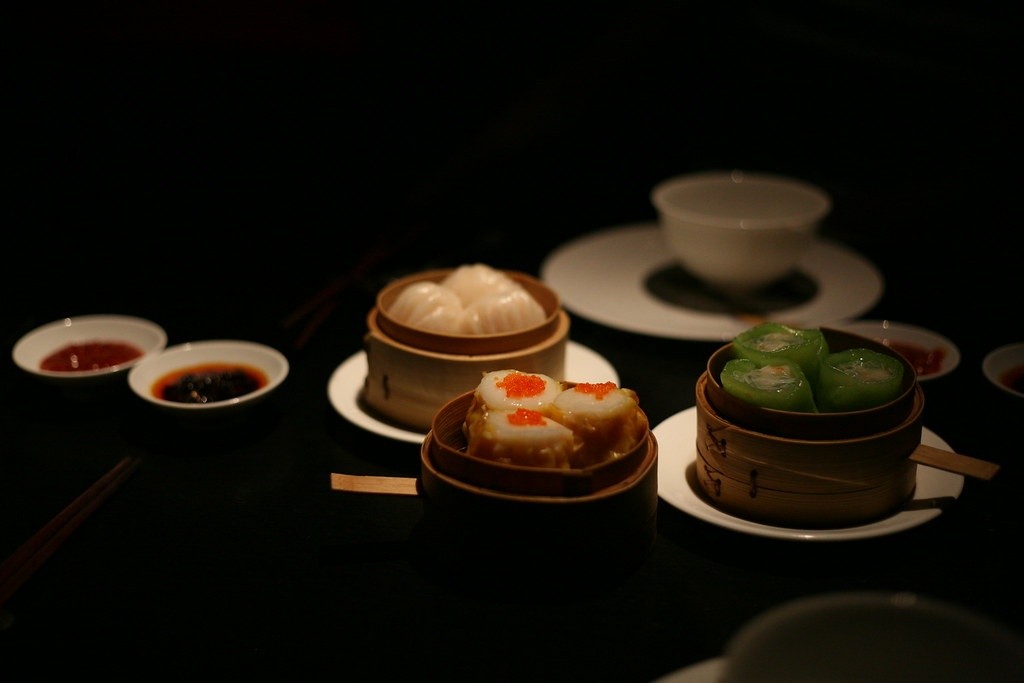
[[0, 442, 154, 609]]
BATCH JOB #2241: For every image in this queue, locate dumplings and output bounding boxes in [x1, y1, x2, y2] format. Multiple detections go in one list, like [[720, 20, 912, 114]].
[[390, 263, 547, 337]]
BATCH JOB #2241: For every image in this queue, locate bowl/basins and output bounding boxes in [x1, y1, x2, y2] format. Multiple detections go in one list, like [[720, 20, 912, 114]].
[[655, 176, 830, 290]]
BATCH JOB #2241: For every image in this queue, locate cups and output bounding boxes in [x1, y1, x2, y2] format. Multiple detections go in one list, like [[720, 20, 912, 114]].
[[727, 596, 1024, 683]]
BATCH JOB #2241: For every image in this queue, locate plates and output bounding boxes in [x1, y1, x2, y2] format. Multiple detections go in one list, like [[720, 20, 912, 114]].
[[13, 313, 170, 381], [125, 339, 289, 414], [982, 343, 1023, 397], [834, 321, 962, 390], [656, 658, 739, 683], [642, 400, 967, 540], [538, 227, 881, 345], [328, 337, 621, 454]]
